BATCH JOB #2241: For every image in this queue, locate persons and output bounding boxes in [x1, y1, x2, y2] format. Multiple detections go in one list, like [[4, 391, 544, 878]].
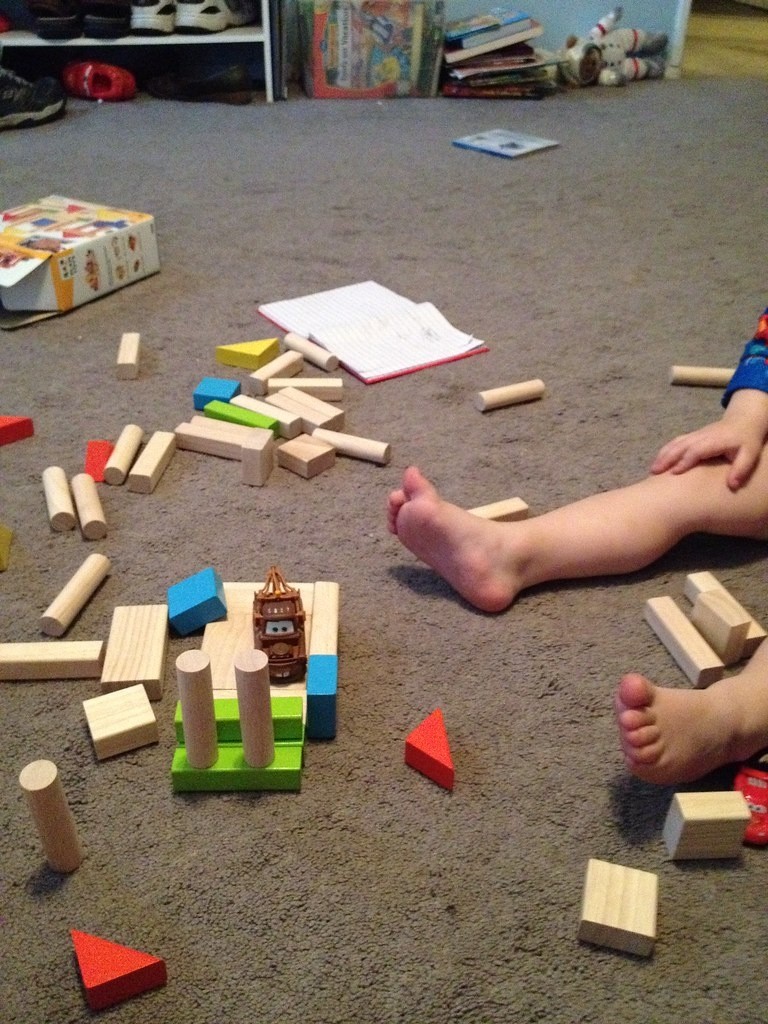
[[385, 304, 768, 786]]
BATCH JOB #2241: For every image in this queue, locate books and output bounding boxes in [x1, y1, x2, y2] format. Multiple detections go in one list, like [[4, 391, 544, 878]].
[[441, 6, 569, 101], [257, 280, 490, 385], [452, 128, 560, 160]]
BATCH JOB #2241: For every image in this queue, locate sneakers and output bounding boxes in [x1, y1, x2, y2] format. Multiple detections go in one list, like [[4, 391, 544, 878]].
[[130, 0, 179, 36], [0, 67, 65, 129], [174, 0, 254, 34]]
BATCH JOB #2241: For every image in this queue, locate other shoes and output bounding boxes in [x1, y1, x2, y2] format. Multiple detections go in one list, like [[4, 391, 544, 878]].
[[31, 0, 84, 41], [81, 0, 131, 39], [63, 62, 136, 101], [143, 56, 252, 105]]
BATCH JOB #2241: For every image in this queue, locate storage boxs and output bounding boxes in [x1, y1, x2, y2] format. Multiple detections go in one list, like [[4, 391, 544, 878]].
[[0, 194, 159, 330], [297, 0, 447, 99]]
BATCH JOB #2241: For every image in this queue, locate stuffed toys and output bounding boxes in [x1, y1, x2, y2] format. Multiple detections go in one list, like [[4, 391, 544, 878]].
[[557, 6, 669, 88]]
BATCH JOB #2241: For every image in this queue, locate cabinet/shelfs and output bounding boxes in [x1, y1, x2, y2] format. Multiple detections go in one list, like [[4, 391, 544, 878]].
[[0, 0, 274, 104]]
